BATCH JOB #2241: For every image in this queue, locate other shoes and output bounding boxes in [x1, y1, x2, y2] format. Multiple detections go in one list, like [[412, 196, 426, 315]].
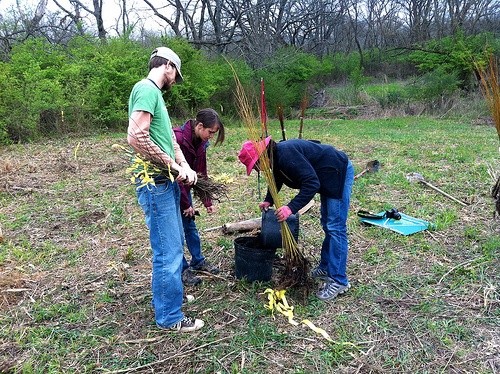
[[193, 260, 219, 275], [181, 269, 201, 286]]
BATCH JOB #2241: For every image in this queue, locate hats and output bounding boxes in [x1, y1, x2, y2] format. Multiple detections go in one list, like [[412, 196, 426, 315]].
[[239, 136, 272, 176], [150, 47, 183, 82]]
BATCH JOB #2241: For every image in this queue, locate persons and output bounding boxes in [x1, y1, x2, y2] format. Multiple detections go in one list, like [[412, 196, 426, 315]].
[[127, 47, 204, 332], [172, 108, 225, 286], [237, 136, 354, 302]]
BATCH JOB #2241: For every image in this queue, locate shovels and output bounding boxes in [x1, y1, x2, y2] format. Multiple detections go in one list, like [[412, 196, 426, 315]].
[[406, 172, 467, 207], [354, 159, 380, 180]]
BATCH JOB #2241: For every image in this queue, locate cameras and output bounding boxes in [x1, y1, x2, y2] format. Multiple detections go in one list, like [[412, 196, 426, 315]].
[[387, 208, 401, 220]]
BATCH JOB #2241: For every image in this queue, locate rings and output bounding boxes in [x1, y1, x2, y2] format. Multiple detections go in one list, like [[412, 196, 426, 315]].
[[281, 215, 283, 218]]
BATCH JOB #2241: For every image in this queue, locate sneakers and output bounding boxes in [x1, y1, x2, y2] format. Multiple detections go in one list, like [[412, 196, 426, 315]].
[[170, 317, 204, 332], [182, 294, 194, 305], [310, 267, 328, 277], [315, 277, 351, 300]]
